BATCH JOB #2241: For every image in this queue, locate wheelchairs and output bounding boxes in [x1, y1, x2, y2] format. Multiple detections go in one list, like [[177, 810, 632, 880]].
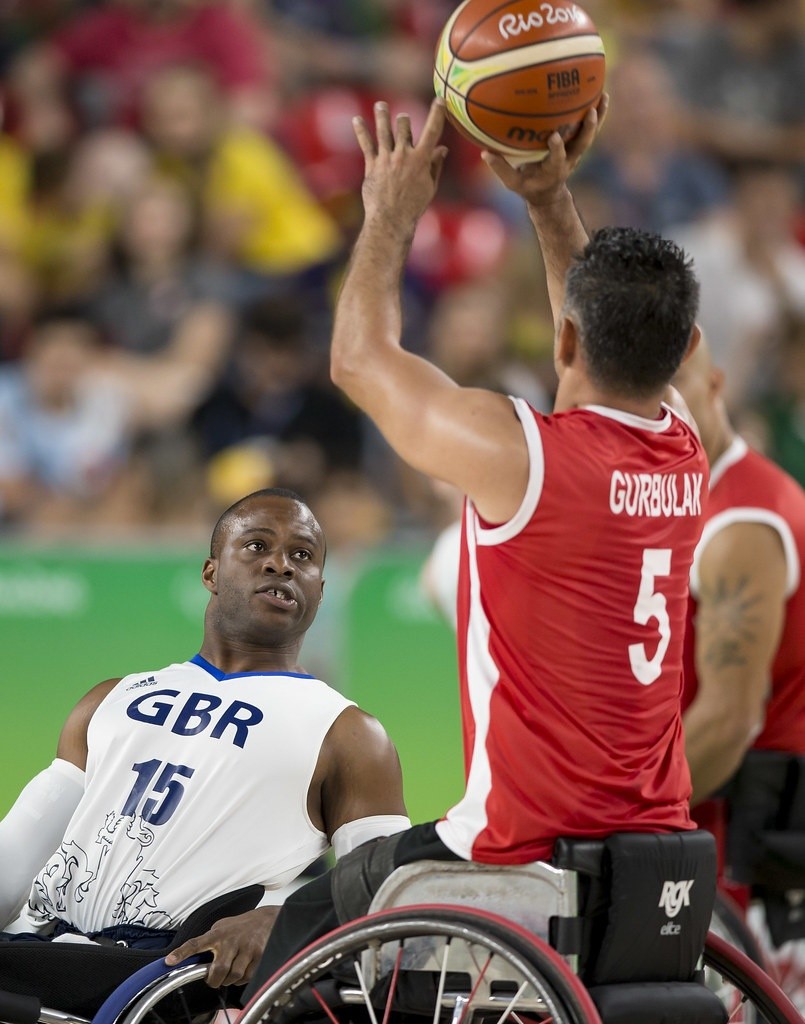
[[40, 746, 805, 1024]]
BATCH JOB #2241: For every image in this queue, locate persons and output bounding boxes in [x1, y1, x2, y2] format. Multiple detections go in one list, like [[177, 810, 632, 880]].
[[672, 313, 805, 949], [0, 0, 805, 544], [0, 483, 408, 1023], [235, 92, 711, 1011]]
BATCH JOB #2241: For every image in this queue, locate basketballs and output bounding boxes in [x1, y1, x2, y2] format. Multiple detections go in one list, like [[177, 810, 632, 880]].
[[432, 0, 610, 157]]
